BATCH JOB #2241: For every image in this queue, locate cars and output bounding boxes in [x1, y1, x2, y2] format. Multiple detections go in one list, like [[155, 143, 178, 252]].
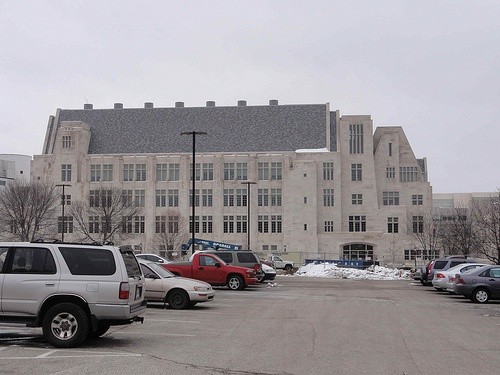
[[266, 256, 295, 272], [453, 265, 500, 306], [259, 257, 272, 267], [92, 258, 216, 310], [254, 263, 276, 282], [136, 253, 169, 265], [432, 262, 500, 294]]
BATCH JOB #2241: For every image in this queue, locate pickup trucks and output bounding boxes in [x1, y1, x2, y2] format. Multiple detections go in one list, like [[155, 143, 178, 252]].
[[158, 253, 257, 291]]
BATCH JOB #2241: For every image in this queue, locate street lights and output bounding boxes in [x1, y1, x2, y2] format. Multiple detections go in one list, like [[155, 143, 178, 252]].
[[55, 184, 72, 244], [240, 181, 258, 251], [179, 130, 207, 257]]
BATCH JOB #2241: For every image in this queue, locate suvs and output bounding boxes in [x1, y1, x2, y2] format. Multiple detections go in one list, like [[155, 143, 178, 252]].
[[196, 245, 263, 277], [0, 238, 148, 348], [420, 259, 478, 287]]
